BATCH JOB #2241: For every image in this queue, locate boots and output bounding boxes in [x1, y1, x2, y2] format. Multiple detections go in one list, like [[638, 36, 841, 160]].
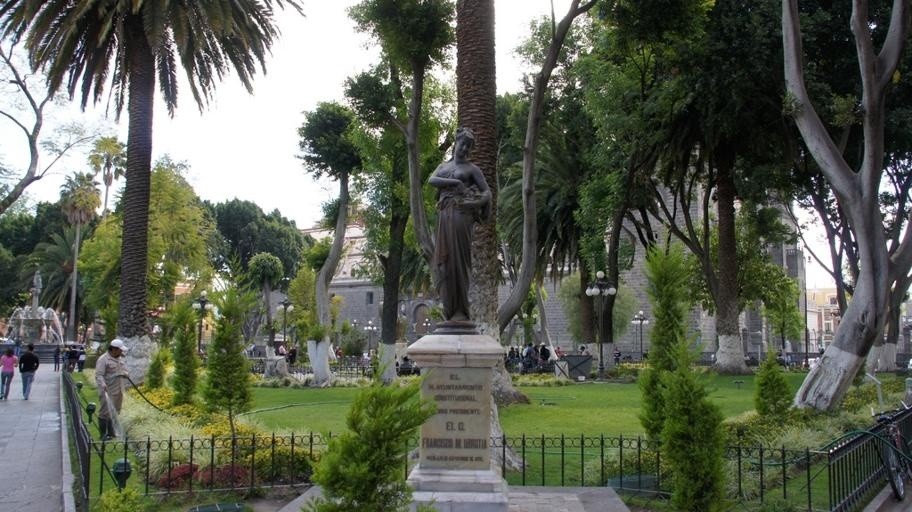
[[99, 417, 119, 440]]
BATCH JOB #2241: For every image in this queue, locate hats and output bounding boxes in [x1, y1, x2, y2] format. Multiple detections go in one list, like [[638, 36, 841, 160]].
[[539, 342, 547, 346], [111, 339, 131, 352]]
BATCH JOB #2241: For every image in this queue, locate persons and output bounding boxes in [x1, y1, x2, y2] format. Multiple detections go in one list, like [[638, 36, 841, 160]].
[[52, 344, 86, 373], [92, 337, 129, 441], [503, 341, 566, 375], [395, 354, 420, 376], [17, 343, 39, 400], [746, 352, 751, 360], [425, 127, 495, 322], [613, 345, 621, 365], [287, 345, 297, 364], [277, 341, 286, 357], [0, 347, 18, 400]]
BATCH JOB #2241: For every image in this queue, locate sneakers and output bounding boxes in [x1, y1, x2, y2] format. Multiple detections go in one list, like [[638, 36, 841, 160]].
[[0, 394, 4, 400]]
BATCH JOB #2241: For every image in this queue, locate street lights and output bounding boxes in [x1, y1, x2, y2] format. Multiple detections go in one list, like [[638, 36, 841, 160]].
[[193, 290, 213, 352], [585, 269, 616, 362], [631, 310, 649, 359], [350, 319, 377, 357], [422, 318, 432, 331], [277, 297, 297, 343]]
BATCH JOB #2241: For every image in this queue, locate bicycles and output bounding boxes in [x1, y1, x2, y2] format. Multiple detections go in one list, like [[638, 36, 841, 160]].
[[871, 403, 912, 501]]
